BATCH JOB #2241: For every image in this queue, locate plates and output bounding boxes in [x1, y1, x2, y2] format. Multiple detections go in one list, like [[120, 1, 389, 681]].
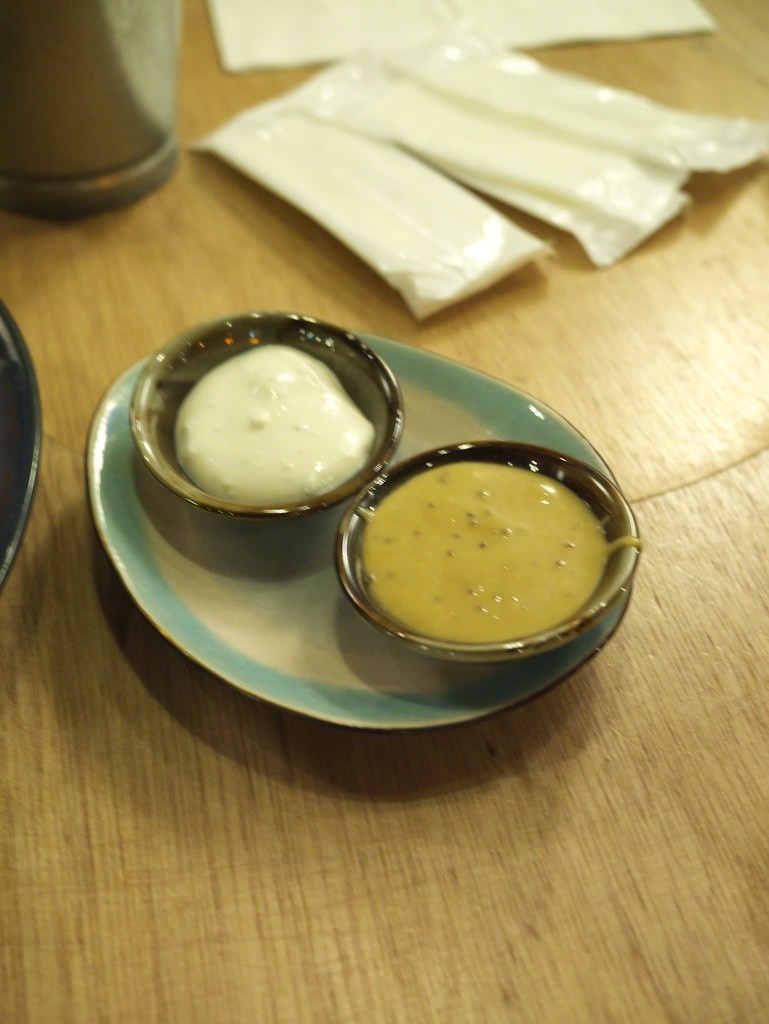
[[0, 300, 44, 593], [85, 327, 638, 732]]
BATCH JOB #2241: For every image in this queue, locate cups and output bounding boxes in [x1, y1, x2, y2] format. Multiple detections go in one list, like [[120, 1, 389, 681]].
[[0, 0, 181, 220]]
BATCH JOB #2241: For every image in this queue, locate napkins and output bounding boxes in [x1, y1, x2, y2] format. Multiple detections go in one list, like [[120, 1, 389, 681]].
[[203, 1, 717, 72]]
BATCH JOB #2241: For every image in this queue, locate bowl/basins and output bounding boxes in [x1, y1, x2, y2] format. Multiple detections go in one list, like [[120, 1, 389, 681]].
[[335, 441, 638, 683], [131, 309, 402, 519]]
